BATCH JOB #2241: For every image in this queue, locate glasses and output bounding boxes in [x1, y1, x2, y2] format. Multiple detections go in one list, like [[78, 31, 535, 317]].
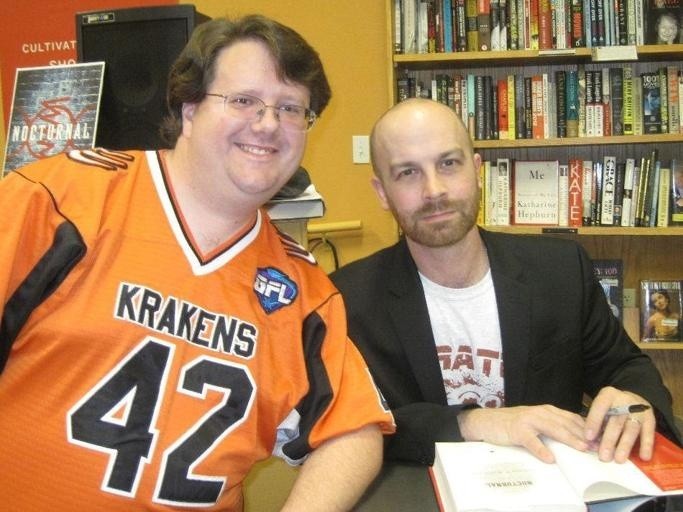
[[205, 91, 317, 131]]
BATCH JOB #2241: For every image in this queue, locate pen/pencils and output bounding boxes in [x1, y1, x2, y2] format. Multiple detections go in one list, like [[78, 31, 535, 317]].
[[607, 404, 651, 416]]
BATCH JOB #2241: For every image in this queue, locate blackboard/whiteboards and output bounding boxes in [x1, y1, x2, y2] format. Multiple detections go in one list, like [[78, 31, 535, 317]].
[[2, 61, 106, 175]]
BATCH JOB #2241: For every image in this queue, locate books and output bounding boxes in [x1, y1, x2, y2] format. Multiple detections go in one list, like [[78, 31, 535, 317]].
[[429, 426, 683, 512], [634, 277, 683, 343], [476, 149, 683, 227], [592, 257, 624, 322], [261, 183, 325, 224], [392, 1, 683, 55], [398, 64, 683, 142]]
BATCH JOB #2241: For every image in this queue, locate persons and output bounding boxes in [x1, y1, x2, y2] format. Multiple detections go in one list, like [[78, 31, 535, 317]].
[[323, 94, 683, 511], [0, 12, 398, 512], [643, 288, 680, 341]]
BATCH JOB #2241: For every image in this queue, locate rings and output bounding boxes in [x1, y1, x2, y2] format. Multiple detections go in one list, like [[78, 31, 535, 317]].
[[627, 417, 642, 428]]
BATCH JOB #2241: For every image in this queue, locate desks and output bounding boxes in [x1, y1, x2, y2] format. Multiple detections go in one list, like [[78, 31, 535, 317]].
[[243, 454, 683, 512]]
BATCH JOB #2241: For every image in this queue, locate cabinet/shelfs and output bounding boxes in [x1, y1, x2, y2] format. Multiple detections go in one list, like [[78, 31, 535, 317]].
[[386, 0, 682, 350]]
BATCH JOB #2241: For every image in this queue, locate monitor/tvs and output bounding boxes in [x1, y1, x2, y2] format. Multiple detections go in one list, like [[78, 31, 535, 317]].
[[75, 5, 213, 151]]
